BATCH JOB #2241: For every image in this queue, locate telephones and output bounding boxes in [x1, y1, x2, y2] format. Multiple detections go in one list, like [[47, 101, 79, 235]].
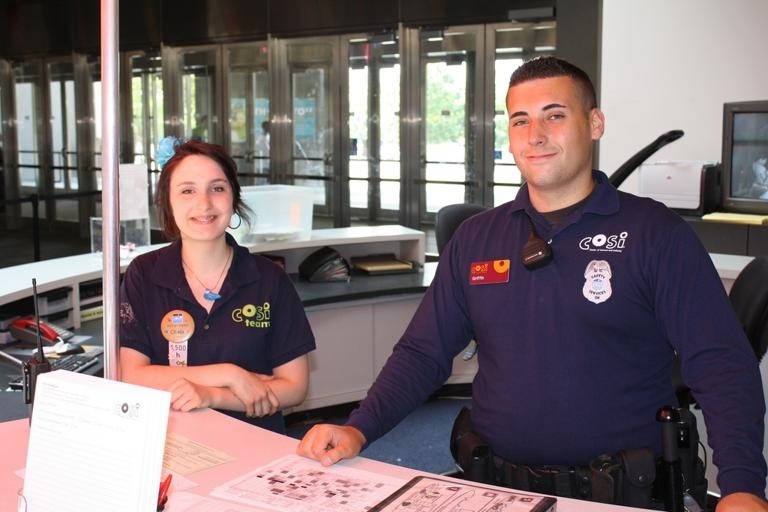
[[9, 316, 74, 346]]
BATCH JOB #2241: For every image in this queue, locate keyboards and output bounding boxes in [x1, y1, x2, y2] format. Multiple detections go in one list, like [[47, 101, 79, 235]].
[[8, 351, 97, 387]]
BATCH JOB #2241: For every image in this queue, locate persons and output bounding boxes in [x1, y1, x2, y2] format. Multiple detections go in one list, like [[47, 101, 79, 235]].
[[119, 140, 316, 436], [298, 55, 768, 512]]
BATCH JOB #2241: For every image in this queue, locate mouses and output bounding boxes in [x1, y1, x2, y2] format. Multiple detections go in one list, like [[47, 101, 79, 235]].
[[56, 344, 85, 353]]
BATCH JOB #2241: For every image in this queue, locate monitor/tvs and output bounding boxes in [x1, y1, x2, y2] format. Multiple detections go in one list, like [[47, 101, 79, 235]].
[[722, 100, 767, 213]]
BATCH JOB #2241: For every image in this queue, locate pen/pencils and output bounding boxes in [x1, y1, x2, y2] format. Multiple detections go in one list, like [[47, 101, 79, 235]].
[[158, 474, 172, 506]]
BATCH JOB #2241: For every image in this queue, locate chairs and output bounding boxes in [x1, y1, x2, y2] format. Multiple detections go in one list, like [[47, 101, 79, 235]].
[[671, 257, 768, 414], [435, 202, 493, 362]]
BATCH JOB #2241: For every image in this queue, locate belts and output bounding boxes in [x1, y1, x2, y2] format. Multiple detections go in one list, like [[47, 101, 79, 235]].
[[490, 458, 589, 496]]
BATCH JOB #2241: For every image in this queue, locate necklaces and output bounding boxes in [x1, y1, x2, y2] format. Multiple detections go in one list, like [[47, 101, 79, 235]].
[[181, 245, 232, 301]]
